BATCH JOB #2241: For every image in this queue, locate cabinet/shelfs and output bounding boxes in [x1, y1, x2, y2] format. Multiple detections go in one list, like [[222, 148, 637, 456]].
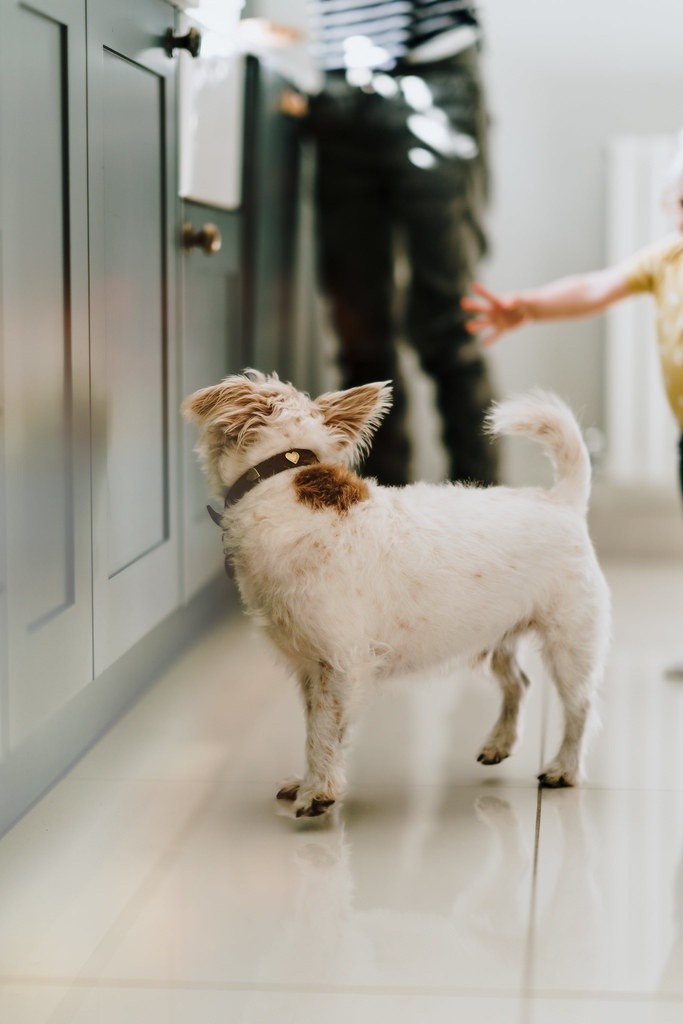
[[0, 0, 342, 833]]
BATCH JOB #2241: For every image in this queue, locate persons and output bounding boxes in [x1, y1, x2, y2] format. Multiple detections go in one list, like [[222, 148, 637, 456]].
[[459, 169, 683, 680], [270, 0, 503, 488]]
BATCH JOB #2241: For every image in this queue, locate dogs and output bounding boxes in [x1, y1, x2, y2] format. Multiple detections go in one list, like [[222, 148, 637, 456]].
[[178, 366, 607, 819]]
